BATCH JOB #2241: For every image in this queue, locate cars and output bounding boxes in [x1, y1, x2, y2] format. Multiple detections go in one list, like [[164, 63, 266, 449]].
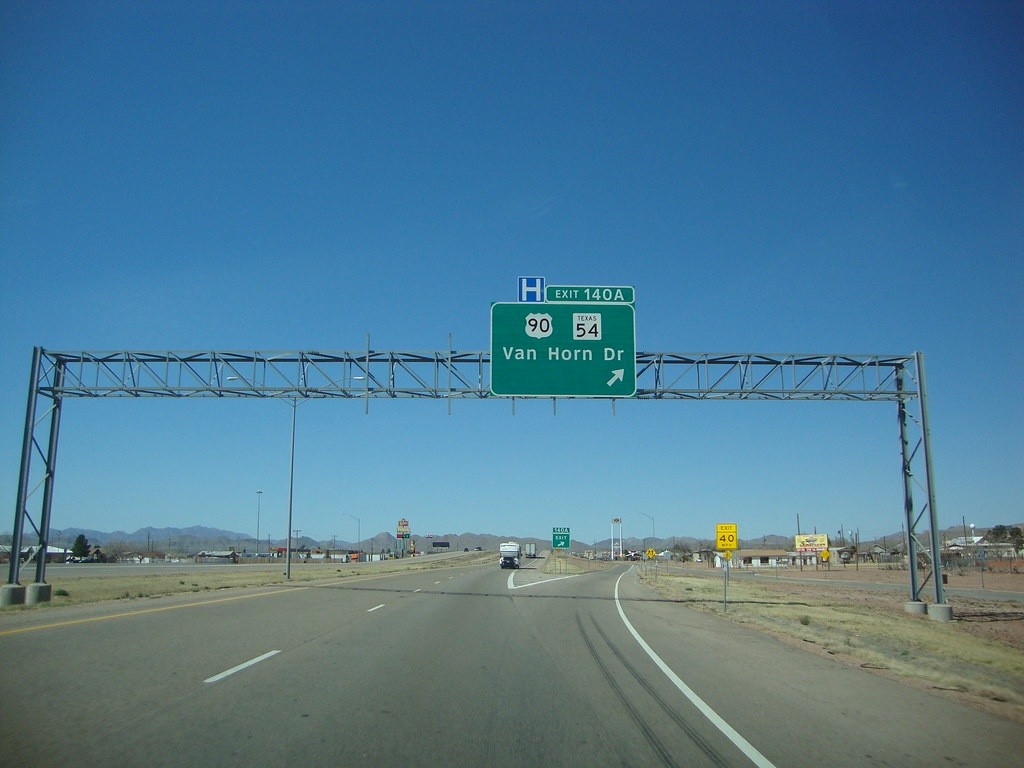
[[464, 548, 468, 552]]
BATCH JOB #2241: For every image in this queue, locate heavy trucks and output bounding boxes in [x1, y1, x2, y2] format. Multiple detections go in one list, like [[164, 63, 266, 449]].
[[524, 543, 537, 558], [499, 541, 521, 569]]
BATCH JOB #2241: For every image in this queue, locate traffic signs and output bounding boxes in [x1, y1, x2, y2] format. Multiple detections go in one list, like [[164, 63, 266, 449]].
[[490, 302, 636, 398], [553, 527, 571, 548]]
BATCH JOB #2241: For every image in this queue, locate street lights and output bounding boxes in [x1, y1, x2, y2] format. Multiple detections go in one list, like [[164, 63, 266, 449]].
[[226, 376, 365, 578], [256, 489, 263, 553], [332, 534, 337, 563], [340, 512, 360, 562], [634, 512, 655, 551], [292, 529, 302, 552]]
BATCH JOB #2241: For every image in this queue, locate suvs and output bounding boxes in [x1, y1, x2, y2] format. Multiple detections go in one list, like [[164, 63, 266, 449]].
[[474, 547, 481, 551]]
